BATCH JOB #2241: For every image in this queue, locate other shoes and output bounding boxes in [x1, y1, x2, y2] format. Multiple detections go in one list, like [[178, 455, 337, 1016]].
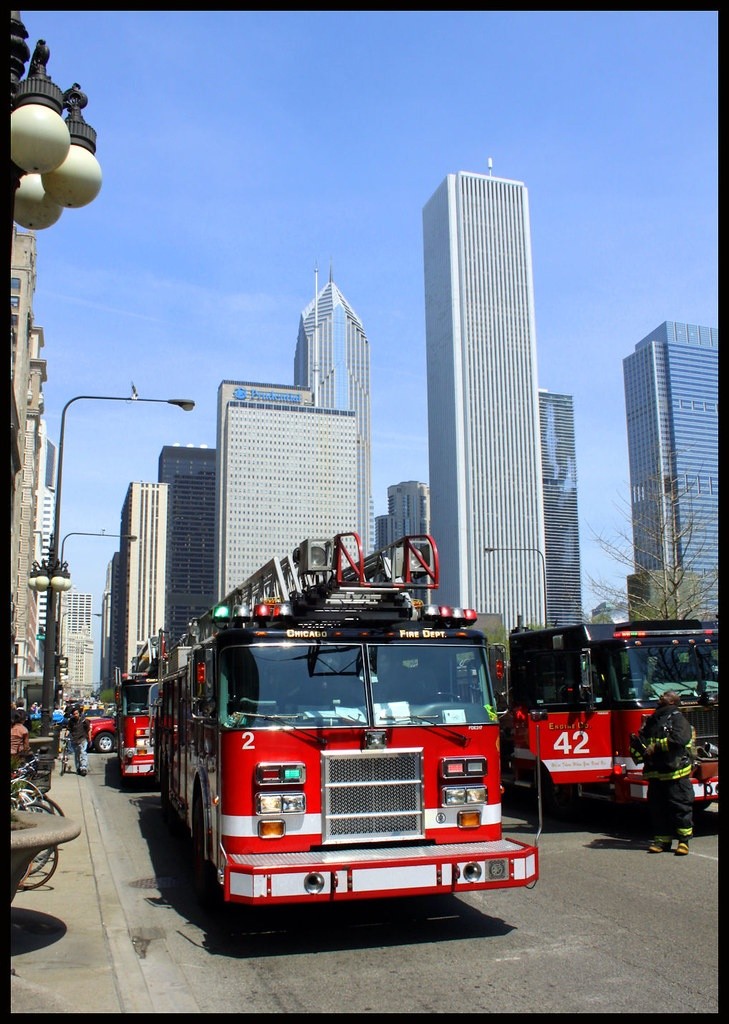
[[676, 843, 689, 855], [81, 769, 87, 776], [649, 842, 672, 852], [77, 768, 81, 775]]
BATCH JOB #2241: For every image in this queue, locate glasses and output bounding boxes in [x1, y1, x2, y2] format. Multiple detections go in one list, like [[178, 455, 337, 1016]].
[[34, 703, 38, 706]]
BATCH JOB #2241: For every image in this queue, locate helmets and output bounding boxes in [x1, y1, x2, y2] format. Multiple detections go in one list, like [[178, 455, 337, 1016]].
[[630, 735, 653, 766]]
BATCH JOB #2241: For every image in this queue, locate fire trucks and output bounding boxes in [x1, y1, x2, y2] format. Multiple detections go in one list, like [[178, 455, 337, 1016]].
[[457, 620, 719, 818], [114, 636, 172, 777], [154, 532, 542, 927]]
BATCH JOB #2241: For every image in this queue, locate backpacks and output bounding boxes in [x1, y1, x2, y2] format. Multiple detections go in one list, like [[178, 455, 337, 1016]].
[[668, 710, 698, 758]]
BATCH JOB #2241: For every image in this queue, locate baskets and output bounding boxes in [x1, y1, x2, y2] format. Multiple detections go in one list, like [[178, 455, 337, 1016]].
[[24, 768, 51, 794]]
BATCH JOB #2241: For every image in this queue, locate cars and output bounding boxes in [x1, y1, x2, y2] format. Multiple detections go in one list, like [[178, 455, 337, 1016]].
[[62, 696, 115, 717]]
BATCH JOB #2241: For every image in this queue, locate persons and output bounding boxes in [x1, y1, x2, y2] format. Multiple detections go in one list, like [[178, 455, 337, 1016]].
[[30, 701, 80, 754], [630, 690, 698, 855], [10, 689, 31, 755], [65, 707, 90, 776]]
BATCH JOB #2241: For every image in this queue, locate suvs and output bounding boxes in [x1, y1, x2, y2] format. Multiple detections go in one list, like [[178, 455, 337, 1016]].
[[85, 707, 118, 753]]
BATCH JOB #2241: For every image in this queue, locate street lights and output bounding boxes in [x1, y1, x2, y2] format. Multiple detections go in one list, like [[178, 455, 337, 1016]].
[[38, 395, 195, 743], [484, 547, 548, 629], [28, 559, 72, 721], [56, 533, 137, 706]]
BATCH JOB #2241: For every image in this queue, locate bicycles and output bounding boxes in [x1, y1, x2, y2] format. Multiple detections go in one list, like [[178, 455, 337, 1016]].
[[10, 757, 65, 890], [58, 737, 70, 775]]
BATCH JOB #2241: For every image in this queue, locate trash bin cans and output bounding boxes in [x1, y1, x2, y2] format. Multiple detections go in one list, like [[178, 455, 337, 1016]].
[[25, 737, 55, 772], [48, 726, 60, 759]]
